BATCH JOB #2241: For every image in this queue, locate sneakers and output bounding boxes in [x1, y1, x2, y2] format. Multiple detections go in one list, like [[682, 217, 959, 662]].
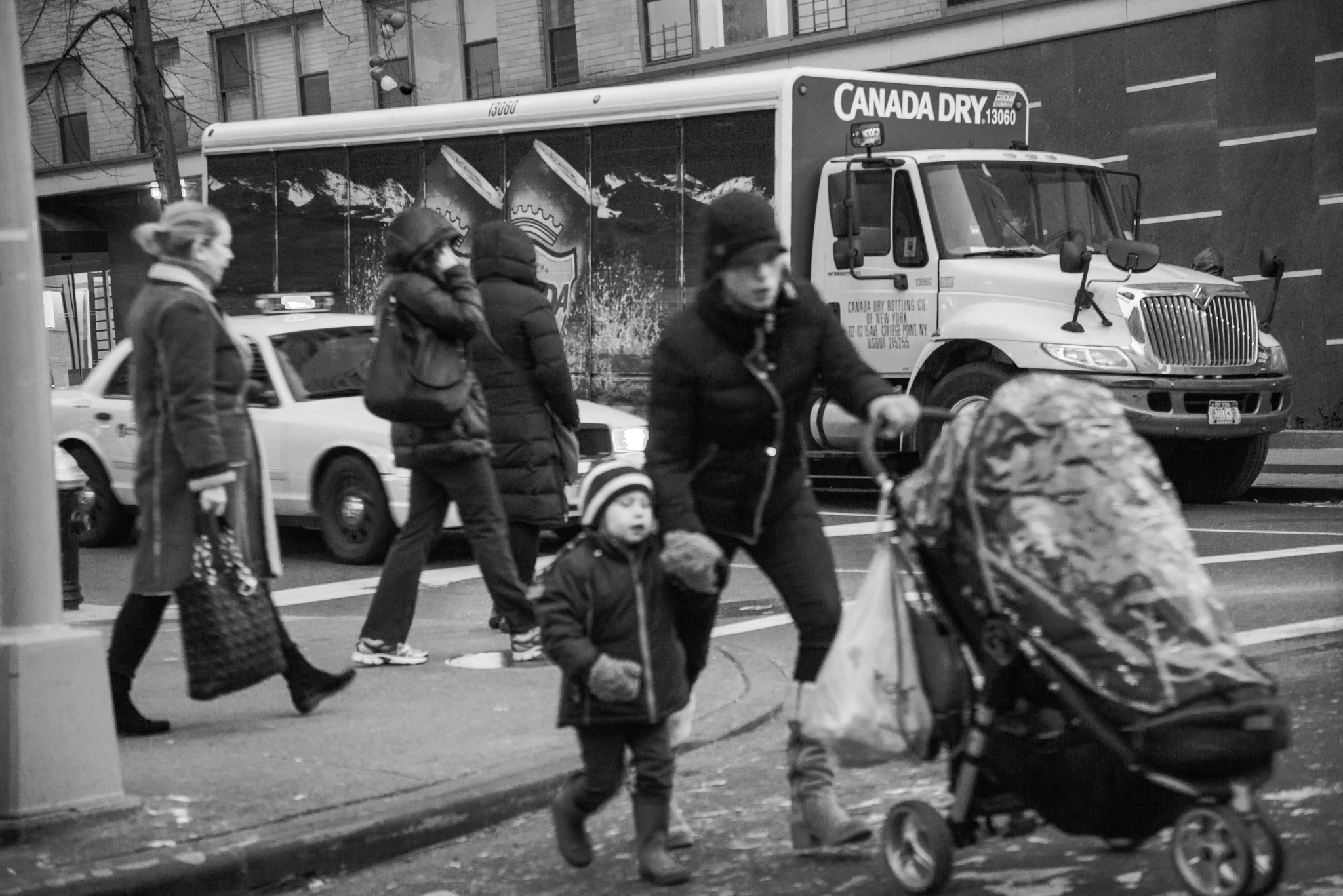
[[510, 627, 545, 662], [352, 633, 430, 664]]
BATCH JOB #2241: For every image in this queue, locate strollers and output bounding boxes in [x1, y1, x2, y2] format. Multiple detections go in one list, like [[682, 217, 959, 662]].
[[859, 368, 1293, 896]]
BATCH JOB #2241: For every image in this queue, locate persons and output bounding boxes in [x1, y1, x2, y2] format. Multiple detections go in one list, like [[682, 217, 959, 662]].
[[640, 194, 924, 850], [468, 222, 580, 631], [539, 460, 713, 885], [998, 211, 1028, 238], [106, 198, 356, 737], [349, 204, 546, 667]]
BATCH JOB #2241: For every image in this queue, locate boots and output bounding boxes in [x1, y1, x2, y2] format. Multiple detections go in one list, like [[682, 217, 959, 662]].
[[663, 692, 696, 847], [551, 768, 605, 870], [784, 679, 872, 848], [631, 791, 687, 884], [280, 644, 355, 714], [104, 647, 171, 736]]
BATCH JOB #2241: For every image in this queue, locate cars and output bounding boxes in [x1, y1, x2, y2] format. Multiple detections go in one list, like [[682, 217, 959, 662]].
[[50, 292, 652, 565]]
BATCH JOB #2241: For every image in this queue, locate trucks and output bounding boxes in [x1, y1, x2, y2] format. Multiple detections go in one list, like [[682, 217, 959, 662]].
[[199, 65, 1293, 506]]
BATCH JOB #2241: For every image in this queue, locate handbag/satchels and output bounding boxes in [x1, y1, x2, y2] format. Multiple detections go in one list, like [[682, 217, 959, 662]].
[[800, 536, 930, 769], [891, 540, 977, 753], [174, 503, 285, 699], [361, 276, 470, 425]]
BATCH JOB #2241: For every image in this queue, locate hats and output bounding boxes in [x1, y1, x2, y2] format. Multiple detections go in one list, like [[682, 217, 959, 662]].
[[577, 458, 654, 526], [700, 188, 787, 278]]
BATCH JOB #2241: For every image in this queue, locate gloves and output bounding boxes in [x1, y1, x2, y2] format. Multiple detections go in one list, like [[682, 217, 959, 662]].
[[588, 652, 642, 703], [659, 528, 715, 586]]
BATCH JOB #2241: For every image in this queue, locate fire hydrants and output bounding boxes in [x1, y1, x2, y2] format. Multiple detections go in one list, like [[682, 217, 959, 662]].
[[52, 445, 89, 610]]
